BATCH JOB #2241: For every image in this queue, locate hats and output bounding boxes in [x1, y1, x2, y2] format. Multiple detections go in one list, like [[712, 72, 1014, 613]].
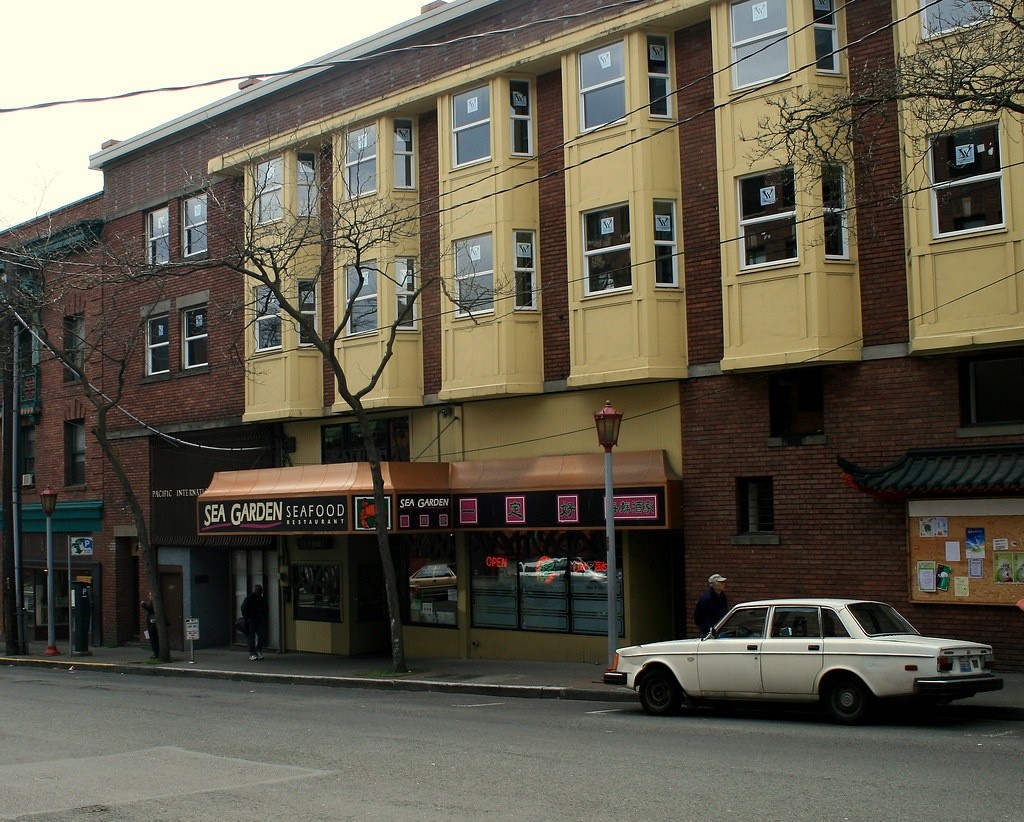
[[708, 573, 727, 583]]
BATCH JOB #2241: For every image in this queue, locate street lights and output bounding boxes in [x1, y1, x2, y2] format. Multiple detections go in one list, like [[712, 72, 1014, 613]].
[[37, 486, 59, 656], [591, 398, 627, 673]]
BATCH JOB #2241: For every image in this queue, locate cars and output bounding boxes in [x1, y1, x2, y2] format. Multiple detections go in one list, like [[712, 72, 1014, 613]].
[[407, 562, 459, 600], [601, 598, 1005, 726], [517, 557, 607, 594]]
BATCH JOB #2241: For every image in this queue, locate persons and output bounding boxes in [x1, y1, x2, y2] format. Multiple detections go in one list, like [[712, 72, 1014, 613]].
[[695, 574, 728, 633], [241, 585, 269, 661], [140, 592, 159, 658]]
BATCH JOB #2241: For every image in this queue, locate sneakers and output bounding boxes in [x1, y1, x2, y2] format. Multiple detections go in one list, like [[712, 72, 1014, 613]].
[[249, 654, 258, 661], [257, 652, 264, 660]]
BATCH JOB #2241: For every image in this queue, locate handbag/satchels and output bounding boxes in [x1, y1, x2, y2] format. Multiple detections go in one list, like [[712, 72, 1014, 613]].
[[234, 618, 248, 637]]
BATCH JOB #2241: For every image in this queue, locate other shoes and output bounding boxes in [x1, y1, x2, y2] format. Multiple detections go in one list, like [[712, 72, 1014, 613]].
[[150, 654, 158, 659]]
